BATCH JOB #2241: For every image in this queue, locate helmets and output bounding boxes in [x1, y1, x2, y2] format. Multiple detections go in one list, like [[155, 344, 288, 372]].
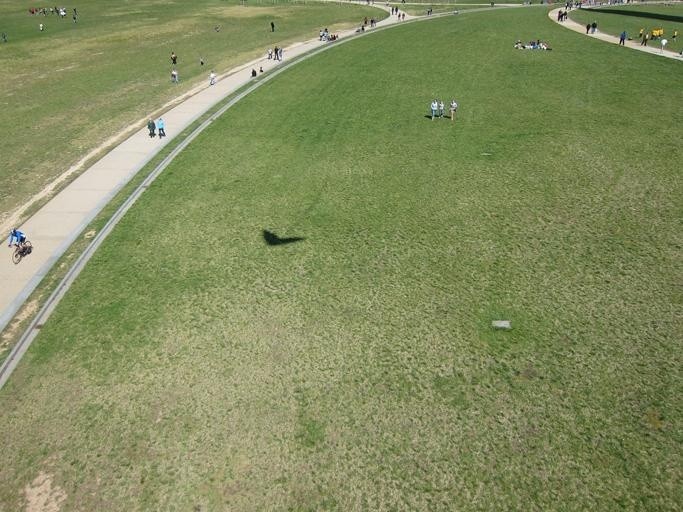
[[10, 228, 16, 235]]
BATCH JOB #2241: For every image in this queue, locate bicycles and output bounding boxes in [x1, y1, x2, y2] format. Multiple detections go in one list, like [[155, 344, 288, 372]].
[[8, 240, 32, 266]]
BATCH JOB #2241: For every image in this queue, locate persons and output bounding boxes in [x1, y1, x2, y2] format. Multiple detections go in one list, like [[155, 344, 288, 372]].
[[157, 117, 165, 138], [273, 46, 279, 61], [169, 68, 179, 86], [512, 38, 553, 51], [591, 20, 598, 35], [556, 10, 568, 23], [430, 98, 438, 122], [618, 30, 626, 46], [490, 1, 494, 7], [27, 5, 77, 33], [209, 71, 216, 85], [521, 1, 634, 10], [448, 99, 458, 122], [277, 46, 283, 62], [317, 0, 433, 42], [585, 22, 591, 34], [637, 27, 678, 55], [146, 116, 156, 138], [7, 227, 26, 254], [270, 21, 274, 33], [267, 46, 274, 60], [438, 100, 444, 119], [170, 52, 177, 71]]
